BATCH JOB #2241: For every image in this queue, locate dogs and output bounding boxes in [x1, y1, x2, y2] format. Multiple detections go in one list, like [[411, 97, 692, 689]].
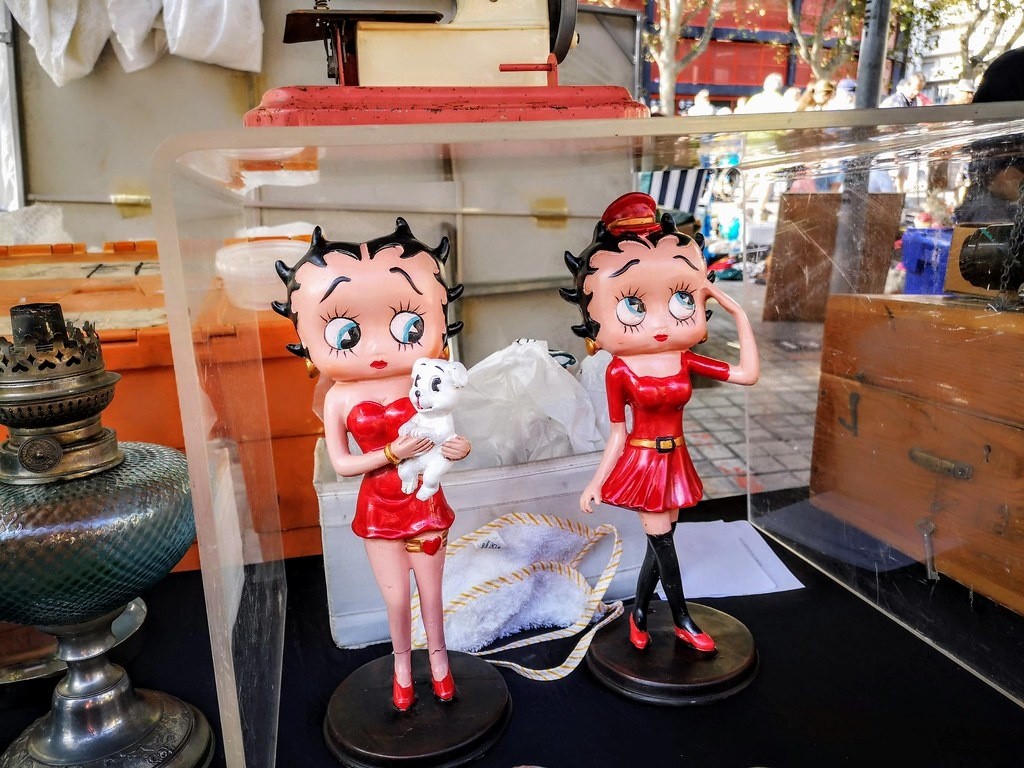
[[398, 357, 468, 501]]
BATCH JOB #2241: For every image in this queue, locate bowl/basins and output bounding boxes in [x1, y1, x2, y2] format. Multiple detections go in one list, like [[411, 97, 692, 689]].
[[215, 239, 310, 310]]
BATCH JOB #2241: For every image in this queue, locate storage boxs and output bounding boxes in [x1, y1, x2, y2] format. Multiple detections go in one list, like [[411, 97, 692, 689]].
[[807, 293, 1023, 618]]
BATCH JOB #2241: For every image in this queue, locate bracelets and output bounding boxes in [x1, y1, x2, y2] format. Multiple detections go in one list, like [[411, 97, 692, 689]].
[[456, 444, 471, 461], [385, 443, 401, 464]]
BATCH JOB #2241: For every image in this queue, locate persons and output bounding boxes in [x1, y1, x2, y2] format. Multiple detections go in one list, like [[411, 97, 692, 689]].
[[559, 193, 760, 652], [271, 216, 472, 709], [687, 74, 858, 221], [869, 71, 973, 207], [968, 46, 1024, 222]]
